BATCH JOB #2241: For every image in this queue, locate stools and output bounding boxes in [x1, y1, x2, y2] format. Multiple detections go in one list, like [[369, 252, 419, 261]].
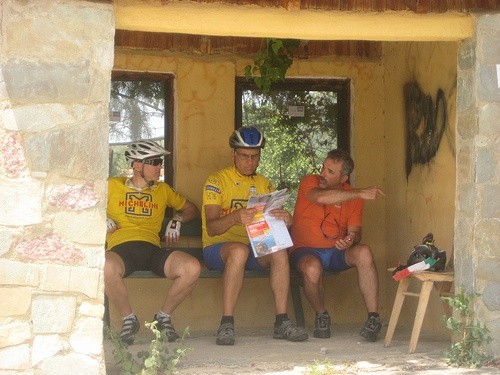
[[384, 268, 454, 353]]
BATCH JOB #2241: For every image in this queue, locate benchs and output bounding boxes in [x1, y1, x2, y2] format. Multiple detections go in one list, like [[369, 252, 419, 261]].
[[104, 217, 305, 339]]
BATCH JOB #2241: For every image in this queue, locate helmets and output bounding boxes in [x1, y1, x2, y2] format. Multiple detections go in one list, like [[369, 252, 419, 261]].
[[229, 126, 265, 148], [407, 233, 446, 272], [125, 139, 171, 167]]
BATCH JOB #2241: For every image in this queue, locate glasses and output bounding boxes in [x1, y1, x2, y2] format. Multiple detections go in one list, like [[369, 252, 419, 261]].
[[138, 159, 163, 166], [320, 212, 339, 239], [236, 152, 260, 160]]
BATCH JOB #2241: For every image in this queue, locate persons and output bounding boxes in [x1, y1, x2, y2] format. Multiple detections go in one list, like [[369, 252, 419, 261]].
[[288, 148, 387, 343], [201, 125, 309, 346], [104, 139, 201, 346]]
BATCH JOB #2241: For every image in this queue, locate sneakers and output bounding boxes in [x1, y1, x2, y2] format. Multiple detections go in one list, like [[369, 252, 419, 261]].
[[153, 314, 179, 342], [314, 313, 330, 338], [274, 319, 308, 341], [216, 319, 235, 345], [120, 316, 140, 345], [360, 316, 382, 338]]
